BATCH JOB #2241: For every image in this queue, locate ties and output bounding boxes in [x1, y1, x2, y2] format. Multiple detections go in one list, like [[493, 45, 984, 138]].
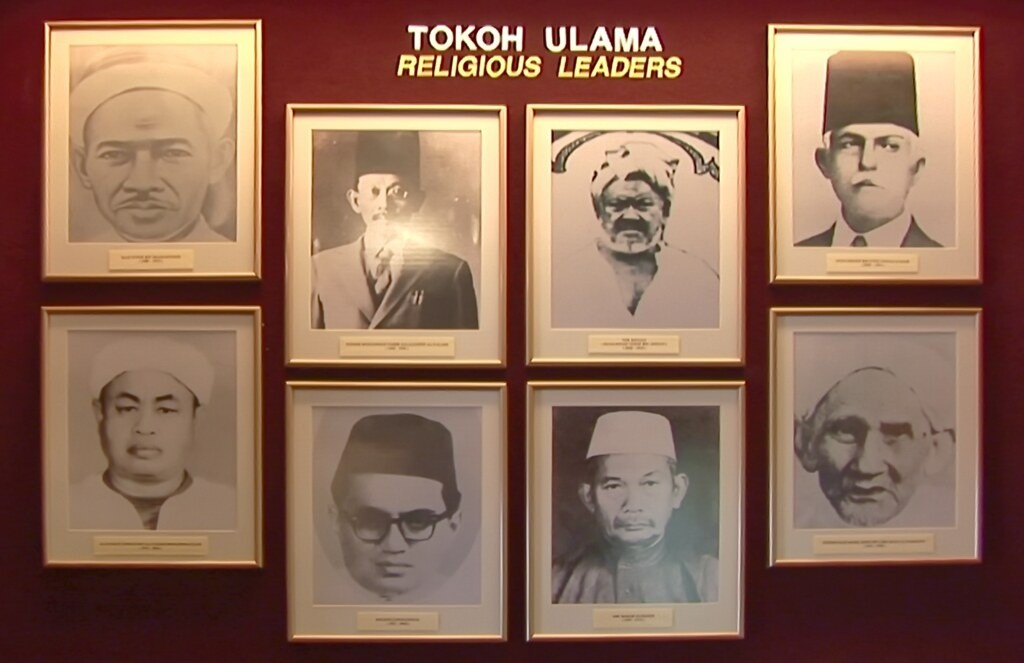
[[374, 249, 392, 301], [853, 235, 868, 247]]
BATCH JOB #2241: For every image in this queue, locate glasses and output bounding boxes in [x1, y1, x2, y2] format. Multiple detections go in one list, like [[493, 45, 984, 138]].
[[345, 506, 451, 543]]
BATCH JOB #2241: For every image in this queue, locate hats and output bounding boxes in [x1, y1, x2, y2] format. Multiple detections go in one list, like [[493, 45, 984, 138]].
[[821, 51, 921, 138], [85, 333, 214, 405], [69, 50, 234, 146], [585, 409, 678, 462], [353, 131, 419, 192], [332, 414, 459, 514], [794, 334, 957, 433]]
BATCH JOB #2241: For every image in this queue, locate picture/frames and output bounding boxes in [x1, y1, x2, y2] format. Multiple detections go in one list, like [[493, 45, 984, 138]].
[[767, 304, 986, 566], [283, 381, 510, 647], [524, 102, 747, 369], [283, 101, 508, 371], [41, 19, 266, 285], [765, 22, 985, 286], [38, 304, 266, 571], [523, 379, 747, 644]]
